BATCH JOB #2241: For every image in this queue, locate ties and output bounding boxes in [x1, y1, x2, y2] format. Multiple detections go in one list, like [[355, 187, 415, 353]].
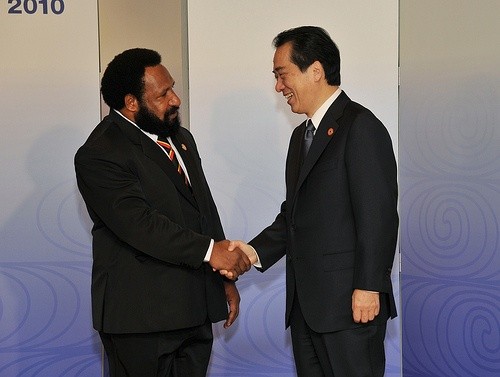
[[299, 119, 316, 173], [157, 135, 193, 194]]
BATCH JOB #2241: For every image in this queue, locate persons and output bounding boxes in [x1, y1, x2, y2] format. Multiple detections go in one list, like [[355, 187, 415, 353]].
[[210, 26, 401, 377], [74, 47, 252, 377]]
